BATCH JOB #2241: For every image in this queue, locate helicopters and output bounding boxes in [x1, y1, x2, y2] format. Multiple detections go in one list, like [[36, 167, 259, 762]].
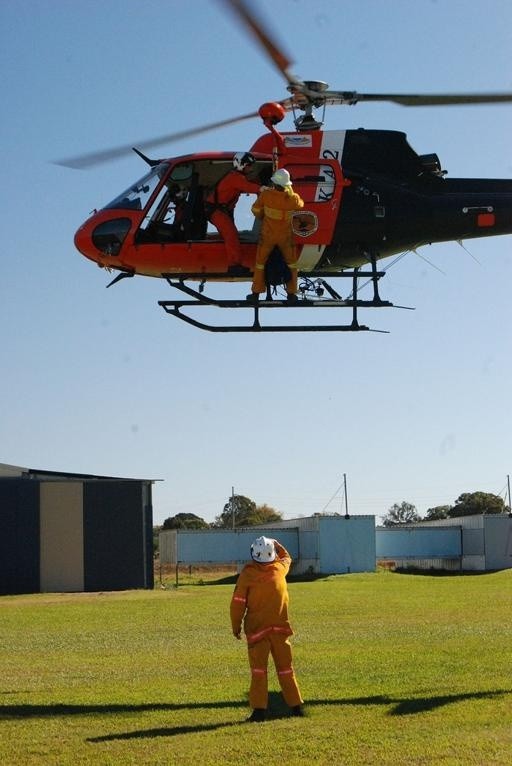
[[52, 1, 511, 334]]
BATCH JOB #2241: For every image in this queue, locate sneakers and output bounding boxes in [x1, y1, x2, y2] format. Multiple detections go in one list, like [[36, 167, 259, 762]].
[[246, 292, 259, 300], [287, 293, 298, 300], [290, 705, 303, 716], [248, 708, 266, 722], [227, 265, 250, 273]]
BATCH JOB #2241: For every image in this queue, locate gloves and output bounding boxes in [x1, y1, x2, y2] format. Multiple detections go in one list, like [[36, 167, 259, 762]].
[[259, 186, 269, 192]]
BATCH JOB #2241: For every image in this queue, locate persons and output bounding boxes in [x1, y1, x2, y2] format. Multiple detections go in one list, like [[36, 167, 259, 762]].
[[168, 184, 193, 225], [230, 536, 304, 722], [246, 168, 304, 300], [204, 152, 270, 273]]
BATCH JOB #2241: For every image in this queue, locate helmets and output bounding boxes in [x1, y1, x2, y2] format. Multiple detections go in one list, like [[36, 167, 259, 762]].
[[271, 168, 293, 186], [251, 536, 275, 562], [232, 152, 255, 171]]
